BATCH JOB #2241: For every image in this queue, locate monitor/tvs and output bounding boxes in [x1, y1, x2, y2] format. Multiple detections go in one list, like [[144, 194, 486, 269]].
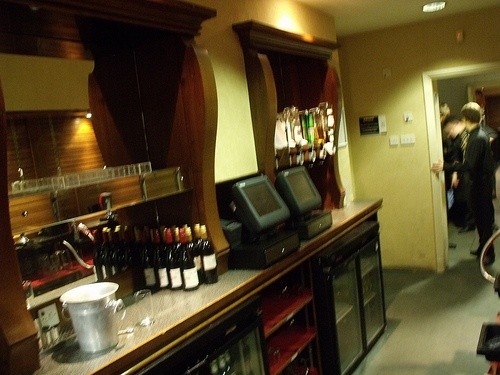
[[274, 166, 321, 222], [232, 174, 290, 237]]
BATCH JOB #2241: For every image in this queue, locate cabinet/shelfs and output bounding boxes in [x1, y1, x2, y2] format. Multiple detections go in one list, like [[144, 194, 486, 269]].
[[141, 216, 388, 375]]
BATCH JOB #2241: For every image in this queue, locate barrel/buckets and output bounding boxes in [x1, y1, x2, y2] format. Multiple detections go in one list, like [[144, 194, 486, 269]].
[[58, 282, 126, 355]]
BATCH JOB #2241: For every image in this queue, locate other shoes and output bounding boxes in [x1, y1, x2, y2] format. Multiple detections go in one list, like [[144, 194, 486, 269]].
[[458, 224, 476, 233], [469, 240, 495, 266]]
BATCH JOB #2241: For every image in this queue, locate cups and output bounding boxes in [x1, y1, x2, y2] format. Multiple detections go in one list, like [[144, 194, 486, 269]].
[[134, 290, 154, 328]]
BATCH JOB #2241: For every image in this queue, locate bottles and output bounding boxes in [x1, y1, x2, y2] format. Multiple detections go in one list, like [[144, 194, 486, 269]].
[[274, 102, 336, 167], [92, 224, 219, 292]]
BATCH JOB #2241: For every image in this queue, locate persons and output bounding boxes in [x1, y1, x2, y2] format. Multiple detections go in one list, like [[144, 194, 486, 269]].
[[438, 100, 500, 266]]
